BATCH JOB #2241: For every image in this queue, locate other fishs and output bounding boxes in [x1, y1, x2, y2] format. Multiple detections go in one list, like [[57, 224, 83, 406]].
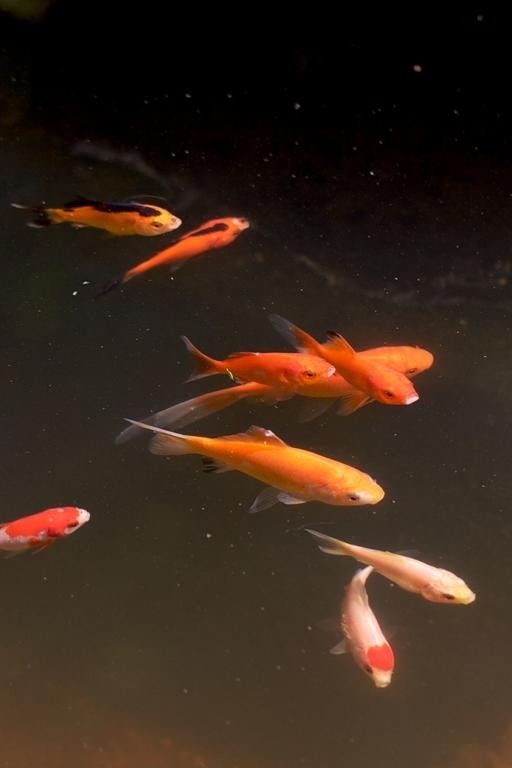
[[90, 215, 252, 302], [8, 193, 183, 241]]
